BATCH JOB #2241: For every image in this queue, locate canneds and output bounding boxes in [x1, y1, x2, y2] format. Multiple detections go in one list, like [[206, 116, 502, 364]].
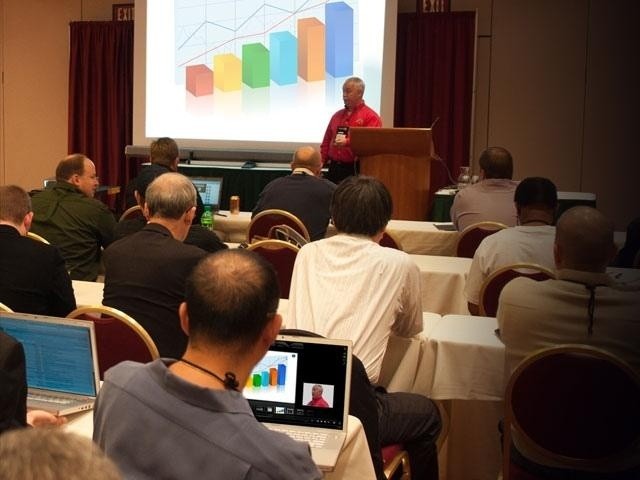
[[229, 197, 241, 214]]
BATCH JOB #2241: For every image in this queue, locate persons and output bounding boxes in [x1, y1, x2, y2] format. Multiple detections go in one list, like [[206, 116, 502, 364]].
[[462, 176, 559, 315], [281, 175, 440, 479], [319, 74, 383, 184], [450, 146, 519, 231], [306, 384, 329, 407], [610, 217, 640, 269], [0, 133, 322, 479], [497, 205, 640, 383]]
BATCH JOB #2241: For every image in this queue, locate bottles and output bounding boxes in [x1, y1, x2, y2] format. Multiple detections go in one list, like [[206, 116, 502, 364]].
[[200, 206, 214, 231]]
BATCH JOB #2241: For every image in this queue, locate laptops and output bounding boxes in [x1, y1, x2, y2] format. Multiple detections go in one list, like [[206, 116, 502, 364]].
[[242, 335, 353, 471], [186, 177, 223, 212], [0, 312, 101, 417]]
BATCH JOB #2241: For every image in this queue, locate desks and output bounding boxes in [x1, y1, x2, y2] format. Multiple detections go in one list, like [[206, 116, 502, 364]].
[[429, 185, 600, 221], [139, 159, 329, 212]]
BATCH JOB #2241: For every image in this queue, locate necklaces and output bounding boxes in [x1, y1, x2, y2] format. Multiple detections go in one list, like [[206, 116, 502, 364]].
[[520, 219, 549, 225]]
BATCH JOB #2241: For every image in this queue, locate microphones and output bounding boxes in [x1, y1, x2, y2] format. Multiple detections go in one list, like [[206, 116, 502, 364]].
[[430, 117, 441, 128]]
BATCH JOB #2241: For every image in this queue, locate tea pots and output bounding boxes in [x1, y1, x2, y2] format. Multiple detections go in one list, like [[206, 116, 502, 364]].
[[457, 166, 473, 190]]
